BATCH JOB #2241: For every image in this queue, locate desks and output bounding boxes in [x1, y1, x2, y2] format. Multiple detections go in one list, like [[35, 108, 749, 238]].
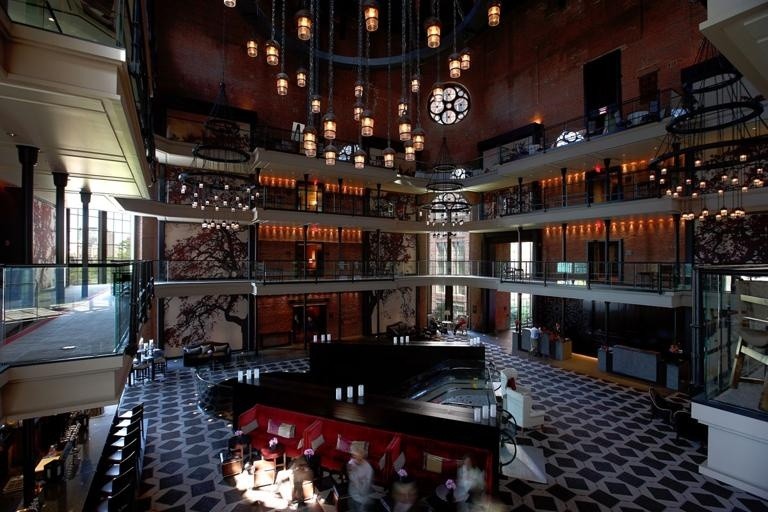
[[441, 320, 452, 328]]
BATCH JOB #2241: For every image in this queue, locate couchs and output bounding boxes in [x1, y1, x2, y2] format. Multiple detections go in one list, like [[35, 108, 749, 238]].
[[182, 339, 231, 367]]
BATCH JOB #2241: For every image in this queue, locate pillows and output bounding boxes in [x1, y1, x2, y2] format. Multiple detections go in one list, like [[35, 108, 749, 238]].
[[183, 343, 228, 356]]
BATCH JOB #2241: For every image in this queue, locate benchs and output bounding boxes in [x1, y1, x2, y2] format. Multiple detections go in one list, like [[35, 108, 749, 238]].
[[236, 403, 497, 502]]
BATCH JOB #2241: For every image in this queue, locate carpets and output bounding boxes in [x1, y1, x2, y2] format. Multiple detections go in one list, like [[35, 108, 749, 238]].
[[500, 441, 549, 487]]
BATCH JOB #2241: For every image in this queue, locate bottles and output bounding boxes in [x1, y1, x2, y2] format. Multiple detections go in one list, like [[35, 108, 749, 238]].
[[473, 375, 478, 384]]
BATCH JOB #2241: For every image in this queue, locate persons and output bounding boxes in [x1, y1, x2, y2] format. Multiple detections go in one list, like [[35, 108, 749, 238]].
[[453, 316, 466, 335], [390, 476, 426, 512], [345, 447, 374, 512], [530, 324, 543, 359], [553, 321, 562, 335], [428, 316, 442, 334]]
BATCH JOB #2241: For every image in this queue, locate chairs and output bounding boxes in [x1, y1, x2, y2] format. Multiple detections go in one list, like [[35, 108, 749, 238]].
[[648, 379, 701, 440], [499, 366, 546, 432], [219, 436, 341, 511], [451, 313, 469, 335], [499, 260, 525, 282], [92, 403, 145, 512], [129, 339, 167, 387]]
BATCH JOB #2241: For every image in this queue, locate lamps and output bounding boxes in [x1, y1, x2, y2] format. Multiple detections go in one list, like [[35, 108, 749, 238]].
[[642, 36, 767, 223], [443, 309, 451, 321], [220, 0, 503, 171]]
